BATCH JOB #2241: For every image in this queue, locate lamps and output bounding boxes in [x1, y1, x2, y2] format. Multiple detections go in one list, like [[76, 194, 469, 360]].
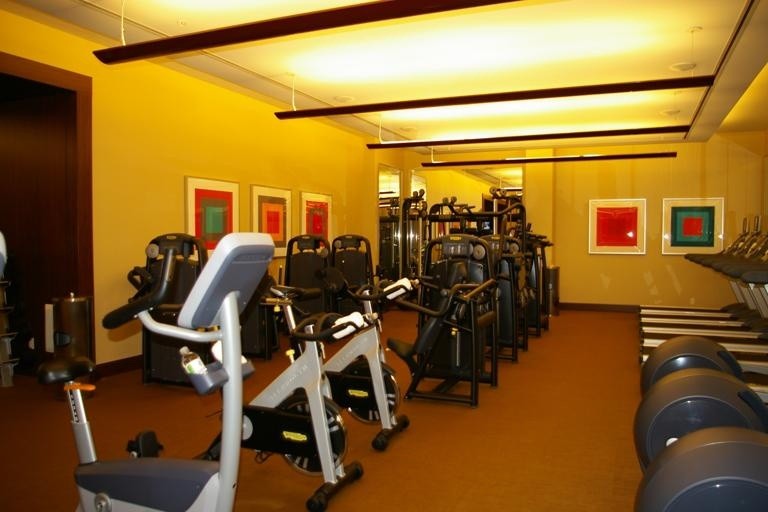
[[275, 75, 713, 120], [92, 0, 505, 65], [420, 147, 676, 165], [366, 120, 690, 150]]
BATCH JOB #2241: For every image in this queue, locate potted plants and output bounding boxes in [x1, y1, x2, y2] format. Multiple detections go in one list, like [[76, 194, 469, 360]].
[[662, 197, 724, 256], [588, 198, 646, 256], [184, 175, 240, 261], [250, 183, 292, 259], [299, 191, 333, 253]]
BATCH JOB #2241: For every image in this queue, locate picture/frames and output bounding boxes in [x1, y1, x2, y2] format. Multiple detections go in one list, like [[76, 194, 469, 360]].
[[299, 191, 333, 253], [588, 198, 646, 256], [662, 197, 724, 256], [184, 175, 240, 261], [250, 183, 292, 259]]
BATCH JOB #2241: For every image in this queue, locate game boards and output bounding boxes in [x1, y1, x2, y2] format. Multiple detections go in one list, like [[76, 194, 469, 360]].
[[640, 215, 768, 510]]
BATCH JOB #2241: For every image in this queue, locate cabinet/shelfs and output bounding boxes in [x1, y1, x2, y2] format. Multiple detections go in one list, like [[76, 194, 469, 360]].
[[360, 311, 379, 328], [410, 279, 419, 288], [178, 345, 208, 376]]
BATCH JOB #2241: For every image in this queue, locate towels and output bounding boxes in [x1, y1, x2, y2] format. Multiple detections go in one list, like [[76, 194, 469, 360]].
[[56, 294, 93, 371]]
